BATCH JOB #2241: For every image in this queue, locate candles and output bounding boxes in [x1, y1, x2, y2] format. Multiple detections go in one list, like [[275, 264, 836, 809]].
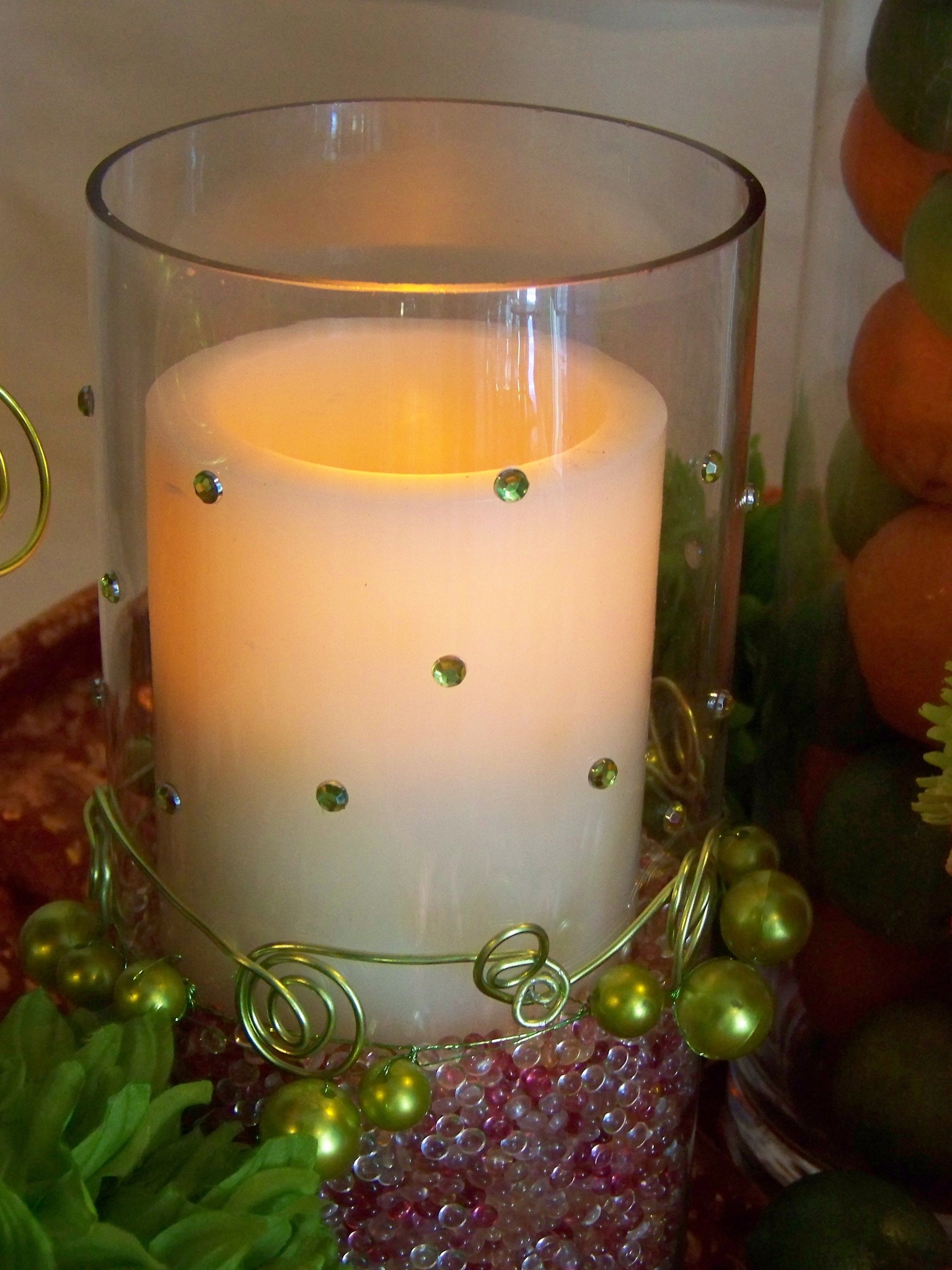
[[137, 315, 670, 1054]]
